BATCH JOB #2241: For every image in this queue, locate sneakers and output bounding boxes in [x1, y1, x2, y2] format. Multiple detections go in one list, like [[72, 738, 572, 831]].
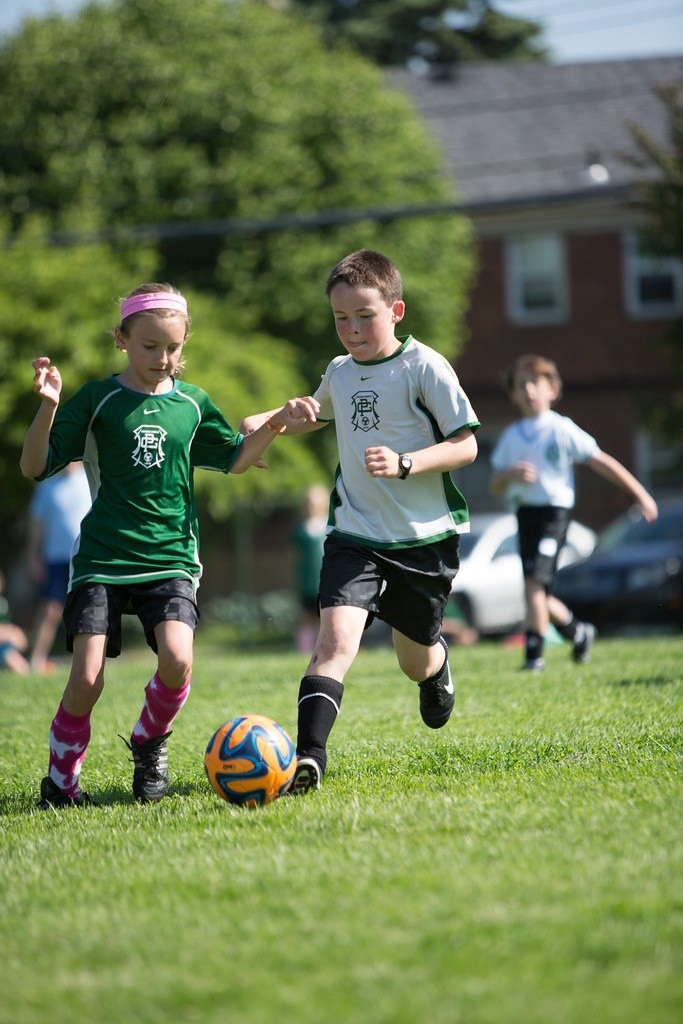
[[289, 757, 323, 793], [418, 635, 455, 728], [119, 727, 171, 801], [38, 774, 94, 810]]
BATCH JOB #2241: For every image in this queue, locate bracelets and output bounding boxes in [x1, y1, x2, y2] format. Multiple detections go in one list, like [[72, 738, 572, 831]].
[[266, 416, 287, 434]]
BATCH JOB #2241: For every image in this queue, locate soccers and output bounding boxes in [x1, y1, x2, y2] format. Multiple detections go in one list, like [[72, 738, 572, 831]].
[[203, 710, 298, 805]]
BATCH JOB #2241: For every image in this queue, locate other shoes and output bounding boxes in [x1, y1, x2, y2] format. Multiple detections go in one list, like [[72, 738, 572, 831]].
[[523, 657, 545, 672], [572, 622, 596, 667]]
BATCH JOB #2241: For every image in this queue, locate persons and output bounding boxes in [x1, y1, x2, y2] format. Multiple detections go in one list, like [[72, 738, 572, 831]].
[[19, 282, 322, 808], [489, 356, 659, 673], [0, 577, 30, 675], [237, 248, 481, 799], [31, 460, 92, 674]]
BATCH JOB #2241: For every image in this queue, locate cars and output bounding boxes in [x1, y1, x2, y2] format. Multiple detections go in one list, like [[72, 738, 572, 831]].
[[363, 507, 596, 652], [547, 496, 683, 632]]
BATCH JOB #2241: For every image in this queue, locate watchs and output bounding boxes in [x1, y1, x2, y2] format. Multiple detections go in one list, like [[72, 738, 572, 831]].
[[397, 453, 412, 480]]
